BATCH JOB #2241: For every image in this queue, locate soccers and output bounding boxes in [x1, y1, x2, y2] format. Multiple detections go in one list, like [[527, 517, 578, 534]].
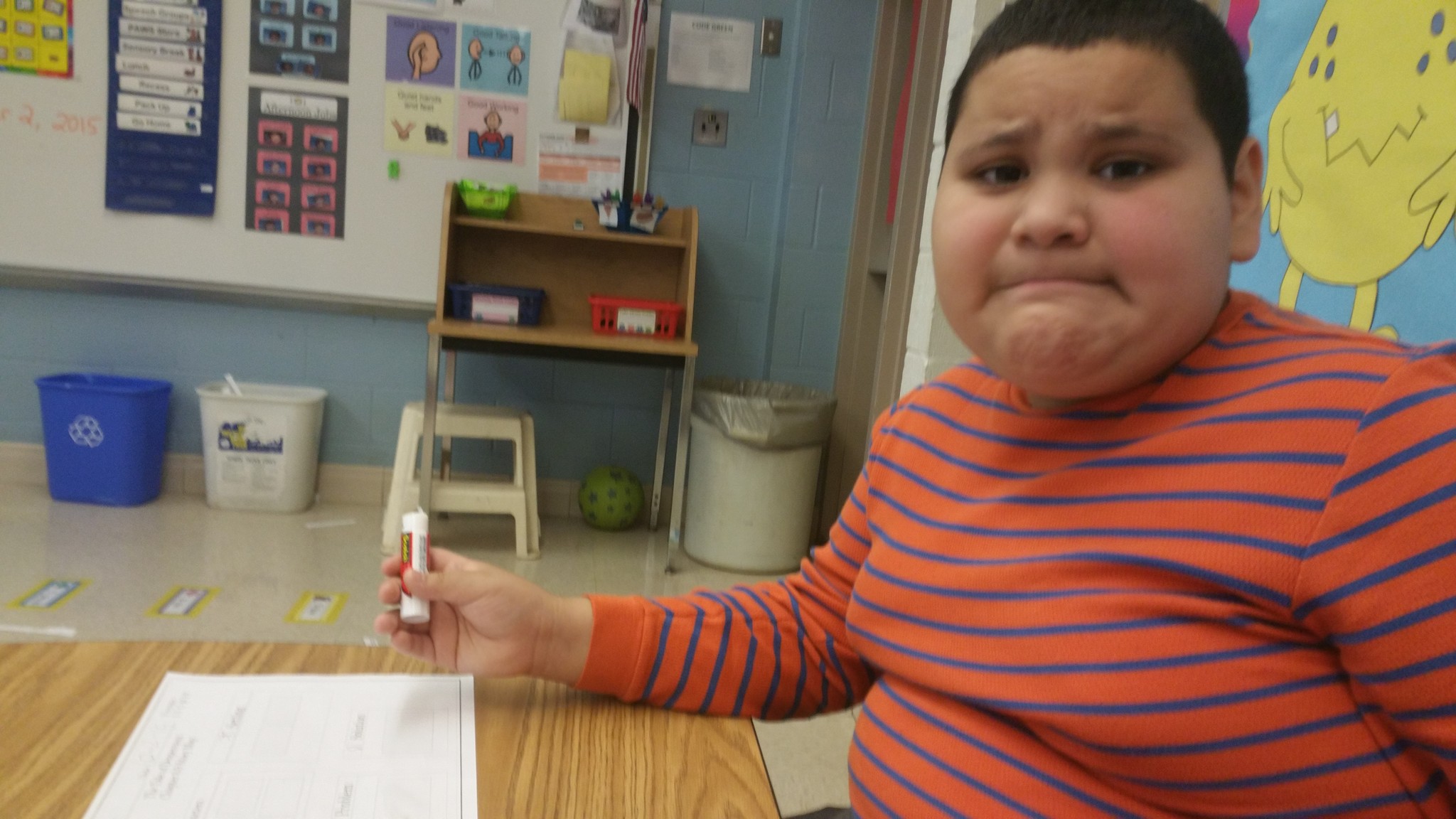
[[576, 464, 647, 529]]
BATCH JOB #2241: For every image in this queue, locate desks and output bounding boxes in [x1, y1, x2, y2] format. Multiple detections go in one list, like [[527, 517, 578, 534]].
[[0, 637, 783, 819], [396, 180, 702, 572]]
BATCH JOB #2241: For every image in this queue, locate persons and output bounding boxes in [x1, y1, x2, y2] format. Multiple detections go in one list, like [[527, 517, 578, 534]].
[[369, 1, 1455, 819]]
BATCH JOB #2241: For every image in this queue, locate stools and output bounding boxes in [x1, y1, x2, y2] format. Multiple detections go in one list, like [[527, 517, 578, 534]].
[[380, 401, 544, 562]]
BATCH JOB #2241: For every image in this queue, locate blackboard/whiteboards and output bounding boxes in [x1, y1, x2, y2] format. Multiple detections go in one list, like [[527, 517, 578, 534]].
[[1, 0, 666, 329]]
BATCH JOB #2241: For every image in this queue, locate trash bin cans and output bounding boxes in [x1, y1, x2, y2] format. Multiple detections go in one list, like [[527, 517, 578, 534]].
[[195, 380, 328, 511], [34, 372, 174, 506], [678, 376, 835, 575]]
